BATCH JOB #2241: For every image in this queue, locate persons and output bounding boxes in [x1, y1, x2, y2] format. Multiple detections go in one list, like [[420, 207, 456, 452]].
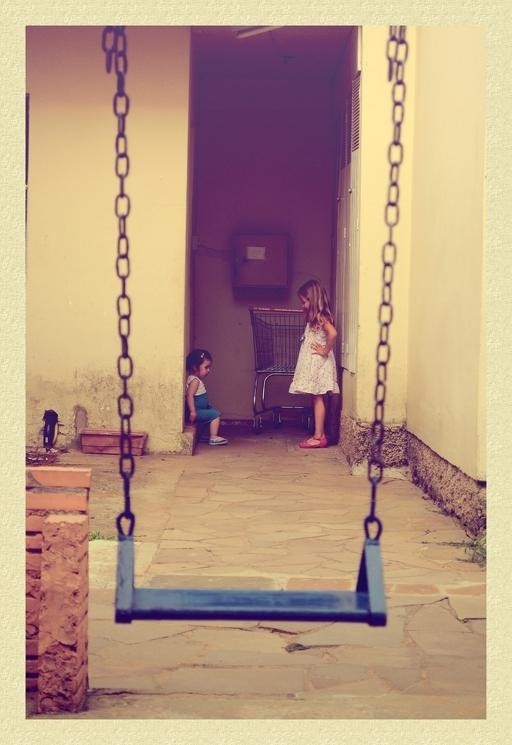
[[184, 347, 229, 446], [287, 278, 341, 448]]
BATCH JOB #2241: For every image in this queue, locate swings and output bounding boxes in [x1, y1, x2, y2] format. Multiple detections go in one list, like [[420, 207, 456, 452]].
[[100, 26, 408, 628]]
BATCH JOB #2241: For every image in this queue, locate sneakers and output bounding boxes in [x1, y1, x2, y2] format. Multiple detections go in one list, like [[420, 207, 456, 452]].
[[209, 436, 228, 446], [198, 433, 209, 441]]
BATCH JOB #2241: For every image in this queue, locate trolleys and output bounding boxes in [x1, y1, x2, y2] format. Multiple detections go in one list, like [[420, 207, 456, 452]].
[[246, 304, 316, 434]]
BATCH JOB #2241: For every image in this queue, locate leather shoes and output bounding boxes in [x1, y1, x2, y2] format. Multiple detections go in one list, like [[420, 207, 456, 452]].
[[299, 436, 328, 449]]
